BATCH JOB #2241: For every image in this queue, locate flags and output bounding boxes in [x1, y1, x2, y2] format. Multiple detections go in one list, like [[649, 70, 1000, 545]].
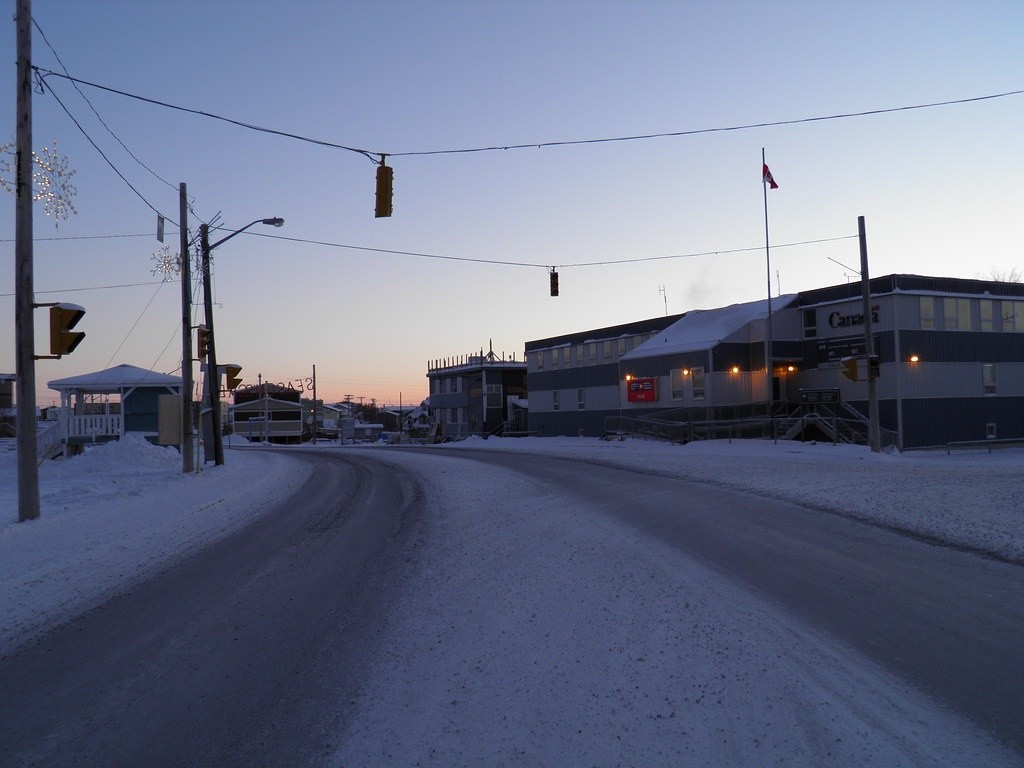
[[762, 164, 778, 189]]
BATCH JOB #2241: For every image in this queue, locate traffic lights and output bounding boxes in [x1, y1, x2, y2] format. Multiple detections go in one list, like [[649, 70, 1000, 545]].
[[841, 357, 857, 379], [227, 364, 243, 389], [869, 356, 880, 378], [550, 272, 558, 296], [50, 303, 86, 354], [198, 324, 212, 358]]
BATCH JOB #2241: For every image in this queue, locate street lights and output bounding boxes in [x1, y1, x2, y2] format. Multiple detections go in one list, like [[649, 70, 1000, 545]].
[[199, 217, 285, 466]]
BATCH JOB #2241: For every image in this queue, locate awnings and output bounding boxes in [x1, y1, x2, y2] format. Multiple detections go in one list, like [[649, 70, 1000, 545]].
[[618, 293, 797, 372]]
